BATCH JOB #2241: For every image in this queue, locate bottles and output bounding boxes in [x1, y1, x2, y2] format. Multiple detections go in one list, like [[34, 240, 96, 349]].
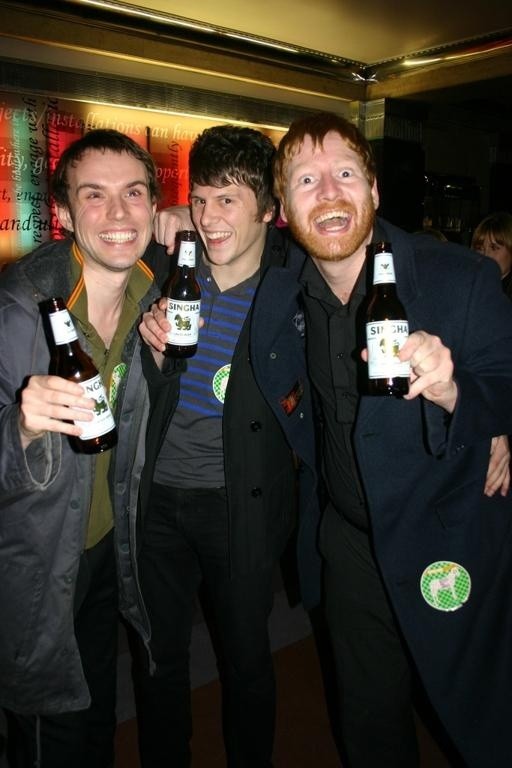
[[164, 229, 205, 359], [359, 243, 412, 396], [41, 297, 124, 455]]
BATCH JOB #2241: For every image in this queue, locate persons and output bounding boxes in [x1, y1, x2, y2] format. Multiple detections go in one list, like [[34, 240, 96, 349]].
[[471, 211, 511, 300], [1, 126, 204, 766]]
